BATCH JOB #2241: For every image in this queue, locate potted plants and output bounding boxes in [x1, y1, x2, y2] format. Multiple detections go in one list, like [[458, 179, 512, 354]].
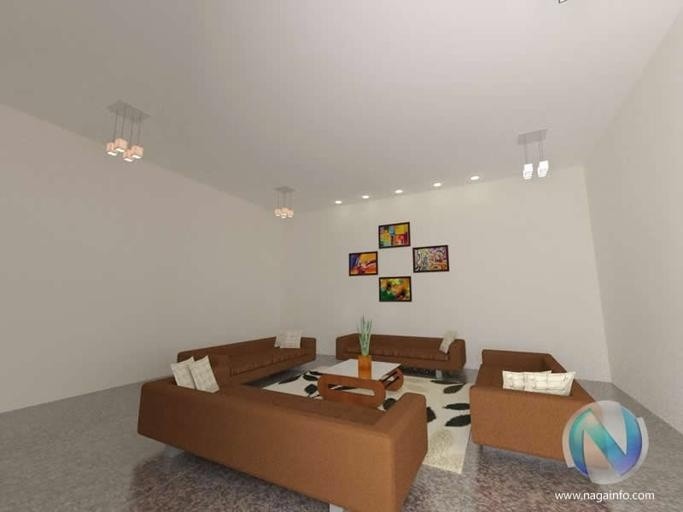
[[354, 313, 373, 379]]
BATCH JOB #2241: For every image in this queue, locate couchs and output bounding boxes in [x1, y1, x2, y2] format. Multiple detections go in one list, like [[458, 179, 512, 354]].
[[137, 378, 428, 512], [335, 333, 466, 379], [176, 334, 315, 386], [468, 349, 603, 487]]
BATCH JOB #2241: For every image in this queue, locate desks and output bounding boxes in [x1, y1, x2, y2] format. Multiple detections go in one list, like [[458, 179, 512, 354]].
[[316, 358, 405, 411]]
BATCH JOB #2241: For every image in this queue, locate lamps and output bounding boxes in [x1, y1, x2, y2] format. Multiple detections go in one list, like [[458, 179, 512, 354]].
[[272, 184, 295, 220], [104, 100, 151, 162], [516, 129, 550, 181]]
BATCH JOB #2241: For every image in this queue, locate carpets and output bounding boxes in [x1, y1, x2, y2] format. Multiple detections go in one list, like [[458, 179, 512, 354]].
[[259, 364, 475, 474]]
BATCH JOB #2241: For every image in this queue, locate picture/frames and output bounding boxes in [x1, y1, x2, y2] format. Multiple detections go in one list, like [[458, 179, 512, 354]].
[[411, 245, 449, 273], [347, 251, 378, 276], [378, 220, 410, 249], [378, 276, 411, 303]]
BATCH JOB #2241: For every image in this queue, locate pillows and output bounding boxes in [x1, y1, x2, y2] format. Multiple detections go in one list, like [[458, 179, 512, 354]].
[[273, 331, 285, 348], [521, 371, 575, 398], [188, 354, 219, 394], [438, 330, 459, 354], [501, 368, 553, 393], [279, 327, 303, 349], [168, 357, 197, 390]]
